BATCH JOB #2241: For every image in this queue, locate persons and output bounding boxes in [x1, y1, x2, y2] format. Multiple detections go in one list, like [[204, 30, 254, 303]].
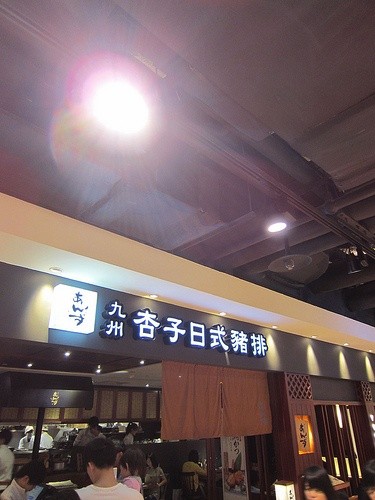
[[0, 429, 15, 492], [349, 468, 375, 500], [182, 449, 208, 500], [0, 460, 46, 500], [58, 438, 145, 500], [301, 465, 348, 500], [72, 416, 139, 446], [112, 439, 168, 500], [18, 424, 54, 450]]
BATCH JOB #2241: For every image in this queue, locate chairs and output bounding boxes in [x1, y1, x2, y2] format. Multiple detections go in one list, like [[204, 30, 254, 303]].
[[159, 471, 196, 500]]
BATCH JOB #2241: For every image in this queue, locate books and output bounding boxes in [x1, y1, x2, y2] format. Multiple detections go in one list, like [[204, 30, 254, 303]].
[[46, 480, 78, 489]]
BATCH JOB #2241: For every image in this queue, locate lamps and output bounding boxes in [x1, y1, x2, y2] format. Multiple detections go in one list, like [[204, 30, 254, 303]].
[[267, 198, 287, 232], [268, 235, 312, 272]]
